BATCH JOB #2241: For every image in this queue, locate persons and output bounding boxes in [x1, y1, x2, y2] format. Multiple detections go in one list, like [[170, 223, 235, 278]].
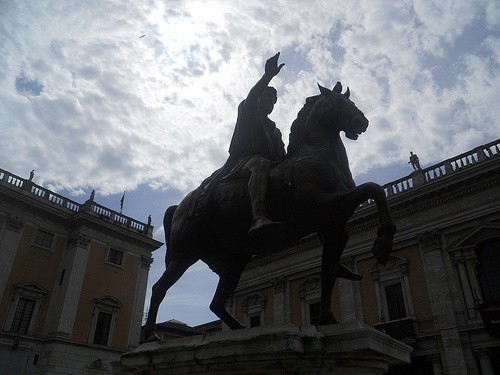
[[219, 51, 366, 281]]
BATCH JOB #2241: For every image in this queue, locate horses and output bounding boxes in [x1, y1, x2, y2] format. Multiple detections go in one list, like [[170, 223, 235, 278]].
[[145, 81, 397, 343]]
